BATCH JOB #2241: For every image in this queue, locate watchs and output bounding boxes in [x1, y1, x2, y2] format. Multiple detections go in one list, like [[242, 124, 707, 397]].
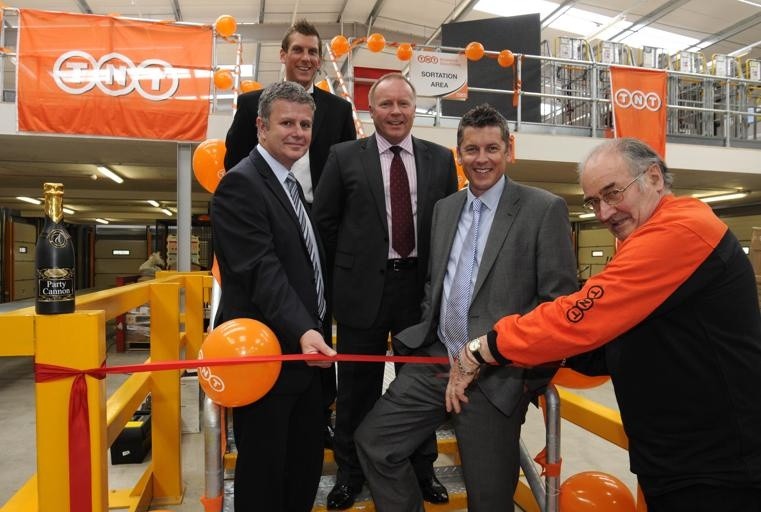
[[468, 337, 485, 365]]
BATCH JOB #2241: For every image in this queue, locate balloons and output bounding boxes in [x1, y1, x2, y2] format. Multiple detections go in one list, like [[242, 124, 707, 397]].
[[367, 33, 385, 53], [241, 81, 264, 94], [558, 471, 637, 512], [214, 71, 234, 91], [398, 43, 414, 61], [465, 42, 485, 62], [331, 35, 349, 59], [193, 139, 228, 194], [315, 79, 330, 93], [498, 50, 515, 68], [215, 15, 237, 38], [198, 318, 283, 407]]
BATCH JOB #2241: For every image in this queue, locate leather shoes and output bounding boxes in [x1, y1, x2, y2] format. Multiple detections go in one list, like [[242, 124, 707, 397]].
[[327, 477, 363, 511], [418, 469, 448, 504]]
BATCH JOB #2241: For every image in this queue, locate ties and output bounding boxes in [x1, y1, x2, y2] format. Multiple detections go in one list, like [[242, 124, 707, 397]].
[[445, 199, 481, 359], [388, 145, 415, 258], [283, 172, 326, 321]]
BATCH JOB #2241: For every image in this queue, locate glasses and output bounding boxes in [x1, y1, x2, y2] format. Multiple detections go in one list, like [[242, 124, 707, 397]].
[[582, 172, 647, 212]]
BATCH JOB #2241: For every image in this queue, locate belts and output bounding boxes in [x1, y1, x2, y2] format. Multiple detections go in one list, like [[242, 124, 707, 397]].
[[388, 260, 410, 269]]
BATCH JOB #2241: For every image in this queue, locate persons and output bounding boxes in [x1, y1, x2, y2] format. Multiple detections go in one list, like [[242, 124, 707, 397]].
[[445, 137, 761, 512], [223, 20, 357, 451], [311, 72, 460, 512], [354, 101, 578, 512], [211, 81, 337, 512]]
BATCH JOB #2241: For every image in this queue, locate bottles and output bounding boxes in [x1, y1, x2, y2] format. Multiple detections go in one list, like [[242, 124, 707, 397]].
[[34, 183, 76, 316]]
